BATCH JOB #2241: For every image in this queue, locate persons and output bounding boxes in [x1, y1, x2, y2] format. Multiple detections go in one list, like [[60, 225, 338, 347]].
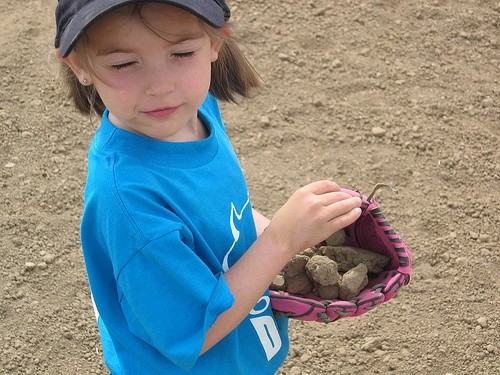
[[55, 1, 363, 375]]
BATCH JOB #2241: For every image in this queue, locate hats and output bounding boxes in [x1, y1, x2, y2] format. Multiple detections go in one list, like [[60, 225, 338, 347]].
[[55, 1, 230, 59]]
